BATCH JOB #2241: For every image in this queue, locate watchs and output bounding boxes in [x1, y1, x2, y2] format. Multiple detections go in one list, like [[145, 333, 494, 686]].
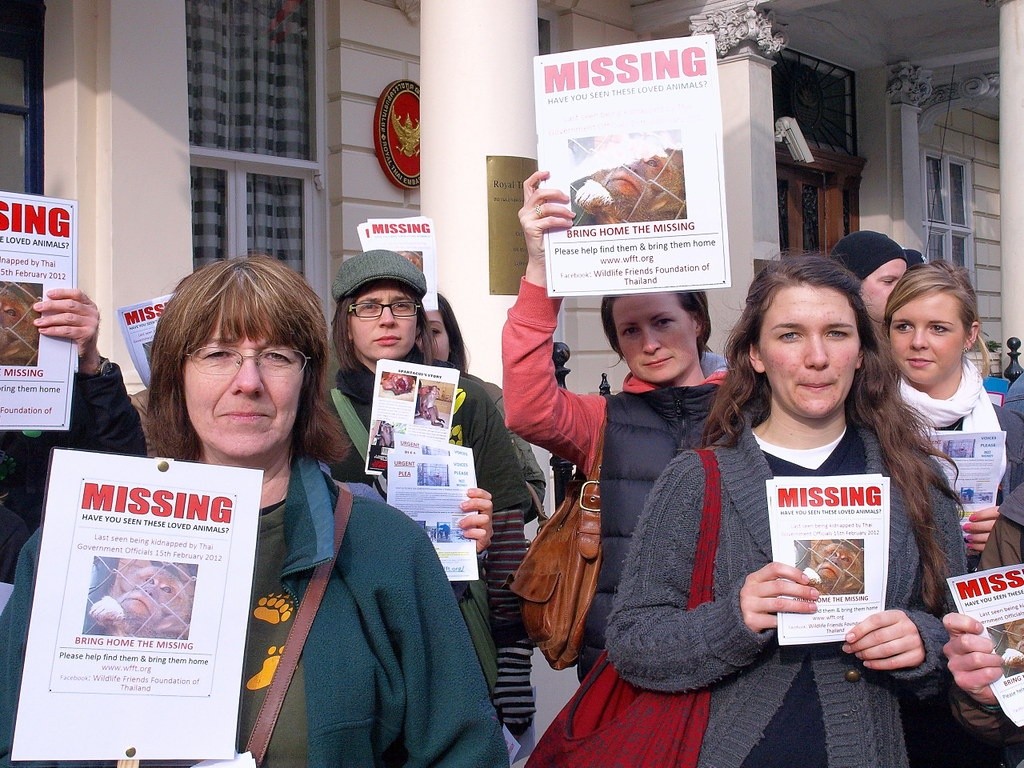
[[86, 353, 114, 377]]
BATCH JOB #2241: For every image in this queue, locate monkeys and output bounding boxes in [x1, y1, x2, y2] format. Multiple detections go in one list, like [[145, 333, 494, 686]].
[[420, 386, 446, 428], [80, 555, 199, 641], [0, 281, 43, 366], [793, 539, 865, 595], [568, 132, 687, 224]]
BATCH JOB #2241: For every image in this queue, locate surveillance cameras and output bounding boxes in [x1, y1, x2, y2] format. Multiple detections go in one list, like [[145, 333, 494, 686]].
[[774, 117, 815, 164]]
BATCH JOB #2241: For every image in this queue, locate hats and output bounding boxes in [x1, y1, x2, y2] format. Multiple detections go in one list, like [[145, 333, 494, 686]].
[[331, 248, 427, 302], [829, 230, 908, 279]]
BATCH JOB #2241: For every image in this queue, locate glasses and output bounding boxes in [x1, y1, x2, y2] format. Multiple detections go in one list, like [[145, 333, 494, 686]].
[[183, 347, 312, 378], [348, 301, 421, 317]]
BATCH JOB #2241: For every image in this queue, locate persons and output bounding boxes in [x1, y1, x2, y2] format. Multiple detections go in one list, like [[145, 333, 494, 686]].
[[825, 229, 912, 334], [0, 252, 514, 768], [312, 250, 538, 768], [410, 287, 551, 526], [932, 478, 1022, 768], [879, 254, 1009, 581], [603, 248, 966, 768], [0, 284, 146, 583], [496, 171, 735, 682]]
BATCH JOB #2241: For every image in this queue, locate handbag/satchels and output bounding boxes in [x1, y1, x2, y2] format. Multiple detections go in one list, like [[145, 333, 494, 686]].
[[509, 393, 614, 670], [521, 450, 721, 768]]
